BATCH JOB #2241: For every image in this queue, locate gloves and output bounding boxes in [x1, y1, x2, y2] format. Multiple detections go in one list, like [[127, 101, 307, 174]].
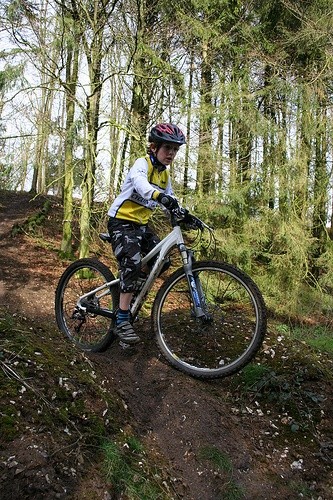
[[156, 193, 177, 208]]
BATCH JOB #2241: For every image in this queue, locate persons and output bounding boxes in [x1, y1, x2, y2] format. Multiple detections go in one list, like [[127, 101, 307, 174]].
[[106, 122, 202, 345]]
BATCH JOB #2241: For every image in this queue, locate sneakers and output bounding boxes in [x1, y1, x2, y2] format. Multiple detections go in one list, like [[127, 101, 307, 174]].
[[112, 320, 140, 344]]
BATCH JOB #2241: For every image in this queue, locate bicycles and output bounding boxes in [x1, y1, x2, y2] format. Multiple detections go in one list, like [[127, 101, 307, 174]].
[[55, 196, 267, 381]]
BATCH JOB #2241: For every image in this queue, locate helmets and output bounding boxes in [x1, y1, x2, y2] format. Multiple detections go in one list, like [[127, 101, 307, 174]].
[[148, 124, 184, 145]]
